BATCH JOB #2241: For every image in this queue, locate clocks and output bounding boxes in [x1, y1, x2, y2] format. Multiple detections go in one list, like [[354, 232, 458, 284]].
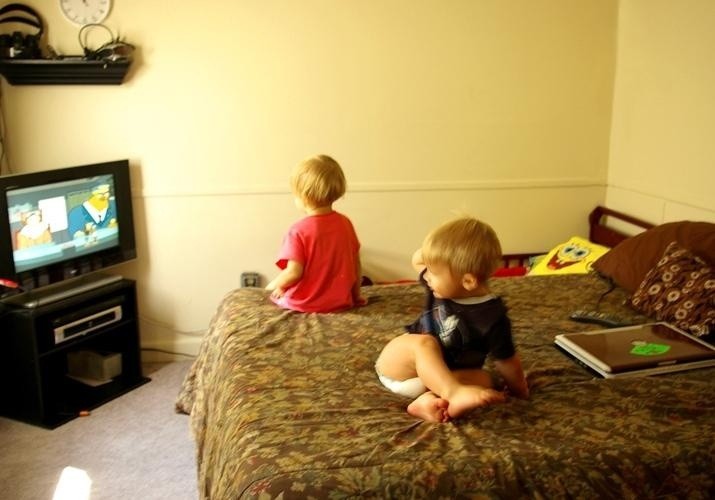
[[57, 0, 114, 28]]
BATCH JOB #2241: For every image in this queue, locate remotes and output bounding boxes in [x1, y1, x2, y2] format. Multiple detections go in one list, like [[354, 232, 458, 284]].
[[570, 309, 633, 327]]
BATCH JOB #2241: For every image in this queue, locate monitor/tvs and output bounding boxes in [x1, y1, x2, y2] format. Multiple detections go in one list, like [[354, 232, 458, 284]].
[[0, 159, 136, 307]]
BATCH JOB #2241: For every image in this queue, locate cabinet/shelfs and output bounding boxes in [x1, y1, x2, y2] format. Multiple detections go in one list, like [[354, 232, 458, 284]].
[[0, 270, 154, 432]]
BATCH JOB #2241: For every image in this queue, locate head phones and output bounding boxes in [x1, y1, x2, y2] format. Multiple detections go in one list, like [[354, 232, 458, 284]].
[[0, 3, 45, 58]]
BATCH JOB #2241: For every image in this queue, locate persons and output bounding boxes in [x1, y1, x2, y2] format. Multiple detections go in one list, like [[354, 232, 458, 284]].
[[266, 153, 370, 313], [374, 217, 533, 425]]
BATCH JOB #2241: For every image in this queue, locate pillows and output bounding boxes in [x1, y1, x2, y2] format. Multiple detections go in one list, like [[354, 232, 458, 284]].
[[588, 218, 715, 347]]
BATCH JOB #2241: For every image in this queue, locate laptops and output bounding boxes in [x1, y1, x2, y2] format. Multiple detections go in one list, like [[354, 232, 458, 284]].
[[555, 321, 715, 374]]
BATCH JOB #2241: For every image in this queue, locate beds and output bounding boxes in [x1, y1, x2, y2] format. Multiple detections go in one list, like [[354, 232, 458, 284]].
[[174, 272, 715, 499]]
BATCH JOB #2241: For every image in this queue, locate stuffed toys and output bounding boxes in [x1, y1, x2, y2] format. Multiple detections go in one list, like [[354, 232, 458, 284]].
[[523, 236, 613, 277]]
